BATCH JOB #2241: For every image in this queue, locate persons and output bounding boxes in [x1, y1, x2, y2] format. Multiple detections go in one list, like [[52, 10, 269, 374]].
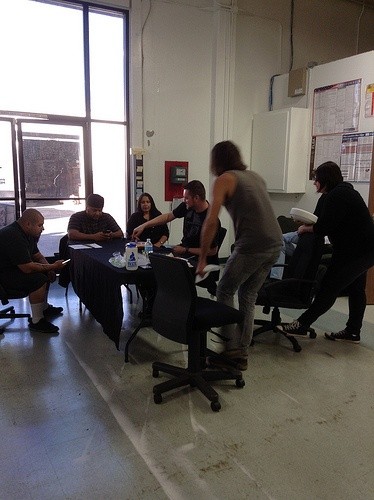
[[0, 208, 66, 335], [126, 192, 169, 255], [196, 141, 282, 372], [196, 218, 303, 295], [67, 193, 123, 239], [277, 161, 374, 343], [131, 180, 221, 266]]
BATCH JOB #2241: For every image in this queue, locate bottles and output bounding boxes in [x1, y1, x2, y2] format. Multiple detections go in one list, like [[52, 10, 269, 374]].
[[145, 239, 153, 257], [126, 242, 138, 270]]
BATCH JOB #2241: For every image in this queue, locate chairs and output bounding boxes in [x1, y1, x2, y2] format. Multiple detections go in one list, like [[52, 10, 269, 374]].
[[0, 281, 31, 334], [147, 253, 247, 412], [54, 233, 71, 297], [250, 233, 326, 352]]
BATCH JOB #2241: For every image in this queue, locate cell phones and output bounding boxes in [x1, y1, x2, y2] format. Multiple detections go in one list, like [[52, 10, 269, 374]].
[[62, 259, 71, 264], [103, 230, 111, 234]]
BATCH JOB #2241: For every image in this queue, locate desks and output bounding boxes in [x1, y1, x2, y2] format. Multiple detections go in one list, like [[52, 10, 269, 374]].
[[65, 239, 210, 363]]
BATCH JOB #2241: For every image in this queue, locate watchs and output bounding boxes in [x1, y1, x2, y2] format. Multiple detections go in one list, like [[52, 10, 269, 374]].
[[159, 241, 164, 244]]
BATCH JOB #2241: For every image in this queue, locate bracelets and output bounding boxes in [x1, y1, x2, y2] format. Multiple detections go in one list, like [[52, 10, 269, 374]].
[[186, 248, 189, 251]]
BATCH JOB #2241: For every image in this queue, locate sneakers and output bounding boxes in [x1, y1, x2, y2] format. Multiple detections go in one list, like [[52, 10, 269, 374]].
[[276, 320, 308, 337], [324, 328, 360, 343], [43, 304, 62, 319], [29, 318, 59, 333]]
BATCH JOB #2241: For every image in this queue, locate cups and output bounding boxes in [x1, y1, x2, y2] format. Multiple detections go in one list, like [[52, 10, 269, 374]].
[[138, 242, 146, 253]]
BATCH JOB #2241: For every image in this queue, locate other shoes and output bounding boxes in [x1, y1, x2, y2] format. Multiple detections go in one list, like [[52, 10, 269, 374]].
[[208, 352, 247, 370]]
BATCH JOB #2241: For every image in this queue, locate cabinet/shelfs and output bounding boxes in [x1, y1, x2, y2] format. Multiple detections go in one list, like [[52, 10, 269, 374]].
[[250, 107, 311, 193]]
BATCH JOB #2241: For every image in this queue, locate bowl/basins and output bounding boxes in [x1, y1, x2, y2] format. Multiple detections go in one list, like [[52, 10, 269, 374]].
[[289, 207, 318, 225]]
